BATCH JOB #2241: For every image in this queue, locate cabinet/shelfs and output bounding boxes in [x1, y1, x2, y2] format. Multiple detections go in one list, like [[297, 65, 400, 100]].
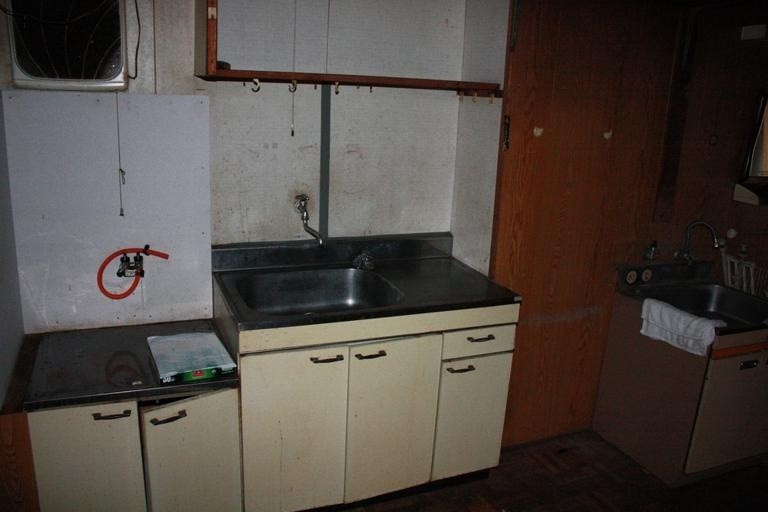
[[455, 3, 766, 445], [238, 333, 443, 512], [589, 291, 768, 489], [430, 327, 508, 488], [29, 382, 244, 512]]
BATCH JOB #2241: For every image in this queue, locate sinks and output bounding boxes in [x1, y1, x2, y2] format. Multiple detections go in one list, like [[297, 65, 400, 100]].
[[638, 280, 767, 335], [232, 267, 407, 311]]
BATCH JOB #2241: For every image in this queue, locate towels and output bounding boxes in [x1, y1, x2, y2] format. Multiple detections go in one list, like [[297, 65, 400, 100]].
[[637, 296, 727, 358]]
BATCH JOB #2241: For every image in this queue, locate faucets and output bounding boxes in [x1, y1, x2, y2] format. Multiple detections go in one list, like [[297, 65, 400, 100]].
[[294, 192, 325, 249], [672, 220, 720, 264]]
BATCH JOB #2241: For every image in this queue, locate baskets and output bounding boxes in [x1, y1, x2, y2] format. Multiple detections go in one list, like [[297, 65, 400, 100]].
[[720, 248, 768, 299]]
